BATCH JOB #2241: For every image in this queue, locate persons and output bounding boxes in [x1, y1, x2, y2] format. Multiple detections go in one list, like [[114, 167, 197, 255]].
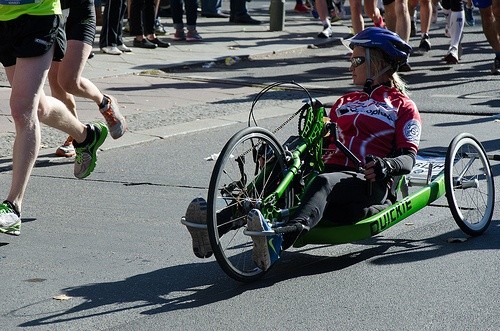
[[200, 0, 230, 18], [129, 0, 170, 49], [99, 0, 132, 55], [48, 0, 127, 157], [185, 27, 422, 272], [169, 0, 203, 40], [154, 0, 169, 34], [229, 0, 261, 25], [296, 0, 500, 75], [0, 0, 107, 235]]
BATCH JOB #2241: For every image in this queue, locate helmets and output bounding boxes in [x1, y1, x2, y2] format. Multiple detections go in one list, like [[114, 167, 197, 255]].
[[346, 26, 413, 65]]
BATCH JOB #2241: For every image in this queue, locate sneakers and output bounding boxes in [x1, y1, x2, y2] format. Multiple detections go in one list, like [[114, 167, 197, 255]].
[[99, 94, 127, 140], [247, 208, 280, 272], [56, 135, 75, 157], [174, 29, 186, 41], [72, 121, 108, 179], [0, 200, 21, 236], [464, 4, 475, 26], [186, 31, 203, 41], [186, 198, 213, 258], [411, 6, 463, 64], [294, 3, 347, 38]]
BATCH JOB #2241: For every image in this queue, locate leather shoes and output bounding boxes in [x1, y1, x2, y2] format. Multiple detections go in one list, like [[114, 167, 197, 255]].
[[147, 37, 170, 47], [133, 38, 158, 49]]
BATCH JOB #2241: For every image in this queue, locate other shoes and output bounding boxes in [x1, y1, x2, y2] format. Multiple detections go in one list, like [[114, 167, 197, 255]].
[[201, 10, 230, 18], [88, 53, 94, 59], [398, 63, 412, 72], [491, 56, 500, 75], [229, 16, 261, 24], [122, 28, 131, 36], [154, 17, 169, 36]]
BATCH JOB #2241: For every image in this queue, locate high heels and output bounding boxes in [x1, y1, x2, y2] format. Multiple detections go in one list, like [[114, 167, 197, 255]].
[[100, 46, 121, 55], [118, 43, 132, 52]]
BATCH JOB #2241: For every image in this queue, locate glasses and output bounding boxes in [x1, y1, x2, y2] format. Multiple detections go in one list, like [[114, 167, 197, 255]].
[[350, 56, 365, 68]]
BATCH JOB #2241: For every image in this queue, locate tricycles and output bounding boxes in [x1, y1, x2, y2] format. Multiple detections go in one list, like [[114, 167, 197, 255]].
[[180, 80, 496, 285]]
